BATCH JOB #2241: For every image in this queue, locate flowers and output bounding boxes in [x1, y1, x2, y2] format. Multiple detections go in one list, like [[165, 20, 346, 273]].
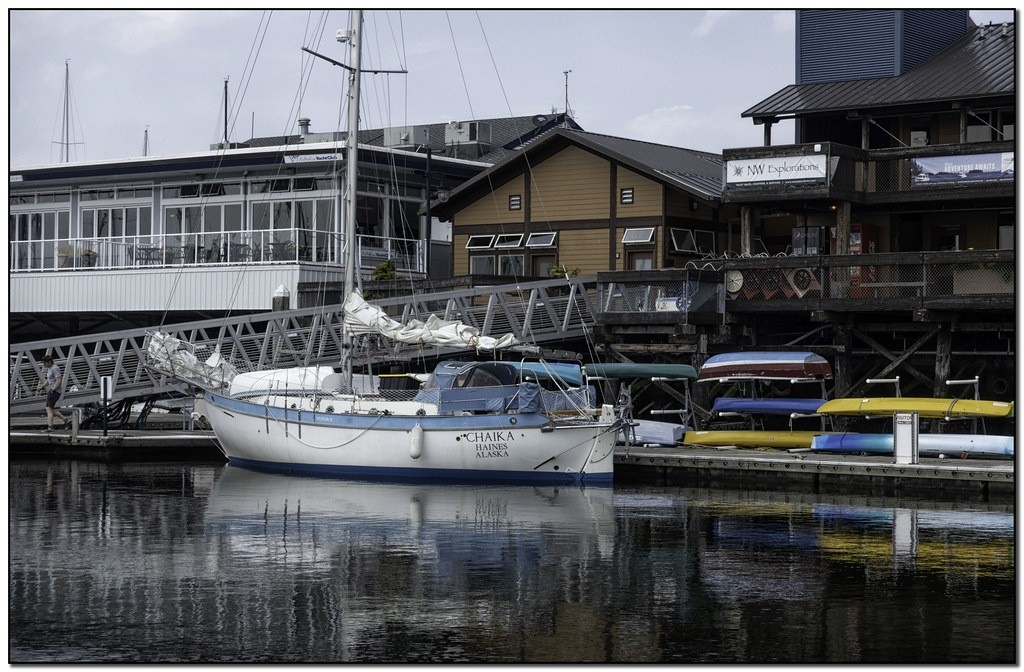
[[82, 249, 98, 257]]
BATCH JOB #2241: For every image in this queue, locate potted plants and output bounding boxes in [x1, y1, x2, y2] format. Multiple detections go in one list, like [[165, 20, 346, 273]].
[[363, 260, 411, 316]]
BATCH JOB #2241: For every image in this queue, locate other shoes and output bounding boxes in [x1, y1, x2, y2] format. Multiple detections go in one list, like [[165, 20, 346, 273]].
[[41, 428, 56, 434], [64, 420, 72, 431]]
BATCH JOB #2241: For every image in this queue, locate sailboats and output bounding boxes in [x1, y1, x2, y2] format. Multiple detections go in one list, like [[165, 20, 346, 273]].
[[201, 8, 641, 488]]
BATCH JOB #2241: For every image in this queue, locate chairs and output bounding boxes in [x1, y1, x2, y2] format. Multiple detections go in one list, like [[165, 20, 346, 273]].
[[128, 240, 303, 260]]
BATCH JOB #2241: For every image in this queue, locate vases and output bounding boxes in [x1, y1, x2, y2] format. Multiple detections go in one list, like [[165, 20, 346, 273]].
[[84, 255, 96, 267]]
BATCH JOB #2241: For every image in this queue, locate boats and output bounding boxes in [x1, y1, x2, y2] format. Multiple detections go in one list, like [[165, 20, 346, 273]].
[[681, 430, 856, 449], [614, 414, 694, 446], [816, 398, 1016, 417], [584, 364, 699, 380], [811, 433, 1017, 459], [694, 351, 834, 383], [715, 397, 828, 417]]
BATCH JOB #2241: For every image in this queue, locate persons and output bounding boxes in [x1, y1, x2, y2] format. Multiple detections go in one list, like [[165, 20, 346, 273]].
[[36, 355, 70, 434]]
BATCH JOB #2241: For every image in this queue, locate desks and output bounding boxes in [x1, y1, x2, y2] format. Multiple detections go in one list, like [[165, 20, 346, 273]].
[[138, 248, 161, 265], [181, 246, 205, 263], [225, 244, 249, 262], [268, 243, 289, 261]]
